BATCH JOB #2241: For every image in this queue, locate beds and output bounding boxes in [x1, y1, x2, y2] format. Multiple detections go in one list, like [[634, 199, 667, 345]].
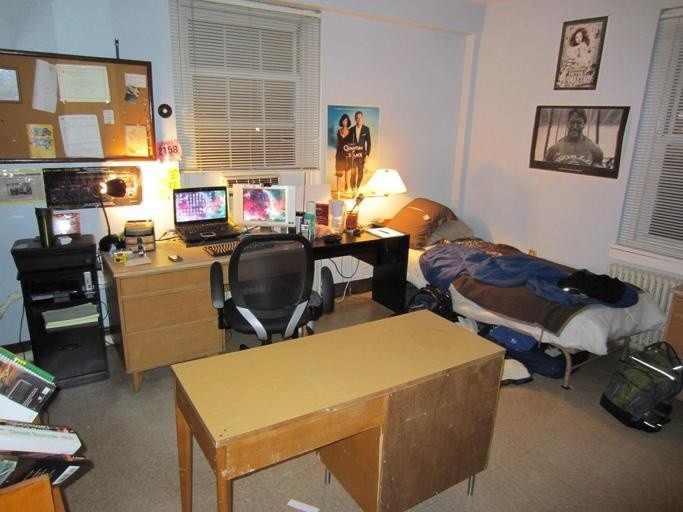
[[387, 197, 666, 390]]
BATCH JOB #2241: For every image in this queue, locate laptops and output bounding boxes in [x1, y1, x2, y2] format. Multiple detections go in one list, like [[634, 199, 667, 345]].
[[173, 187, 241, 243]]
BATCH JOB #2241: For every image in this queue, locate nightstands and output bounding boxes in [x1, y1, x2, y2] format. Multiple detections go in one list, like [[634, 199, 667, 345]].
[[663, 283, 683, 362]]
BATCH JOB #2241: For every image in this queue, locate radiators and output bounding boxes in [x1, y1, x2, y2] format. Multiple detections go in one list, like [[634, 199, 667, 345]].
[[606, 263, 683, 348]]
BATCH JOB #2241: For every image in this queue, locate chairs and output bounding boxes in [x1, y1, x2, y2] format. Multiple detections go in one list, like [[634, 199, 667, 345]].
[[209, 234, 335, 352]]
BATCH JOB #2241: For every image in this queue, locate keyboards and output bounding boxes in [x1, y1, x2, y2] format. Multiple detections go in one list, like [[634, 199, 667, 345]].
[[203, 240, 240, 256]]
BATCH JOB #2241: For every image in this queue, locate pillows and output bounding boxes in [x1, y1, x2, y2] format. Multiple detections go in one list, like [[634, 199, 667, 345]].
[[388, 197, 457, 250], [430, 219, 474, 245]]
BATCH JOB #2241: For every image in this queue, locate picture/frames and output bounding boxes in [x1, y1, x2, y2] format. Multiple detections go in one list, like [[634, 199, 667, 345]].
[[0, 49, 158, 164]]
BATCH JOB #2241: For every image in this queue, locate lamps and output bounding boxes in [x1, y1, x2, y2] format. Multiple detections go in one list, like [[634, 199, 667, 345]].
[[92, 178, 128, 253], [346, 168, 407, 228]]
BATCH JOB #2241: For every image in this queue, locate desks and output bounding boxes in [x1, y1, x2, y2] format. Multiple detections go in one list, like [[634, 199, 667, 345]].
[[307, 228, 410, 316], [98, 237, 305, 393], [169, 309, 507, 512]]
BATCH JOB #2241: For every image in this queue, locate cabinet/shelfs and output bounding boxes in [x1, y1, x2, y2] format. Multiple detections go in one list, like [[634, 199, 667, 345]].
[[11, 234, 110, 390]]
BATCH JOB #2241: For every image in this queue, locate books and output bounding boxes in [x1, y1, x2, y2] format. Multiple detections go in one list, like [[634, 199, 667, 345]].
[[0, 345, 61, 423], [1, 419, 92, 489]]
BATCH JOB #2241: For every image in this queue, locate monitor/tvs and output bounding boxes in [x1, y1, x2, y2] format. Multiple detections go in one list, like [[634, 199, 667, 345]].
[[233, 183, 295, 235]]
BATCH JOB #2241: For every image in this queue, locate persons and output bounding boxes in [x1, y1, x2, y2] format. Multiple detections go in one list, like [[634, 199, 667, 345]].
[[350, 112, 371, 197], [335, 114, 354, 194], [545, 109, 603, 170], [559, 29, 592, 84]]
